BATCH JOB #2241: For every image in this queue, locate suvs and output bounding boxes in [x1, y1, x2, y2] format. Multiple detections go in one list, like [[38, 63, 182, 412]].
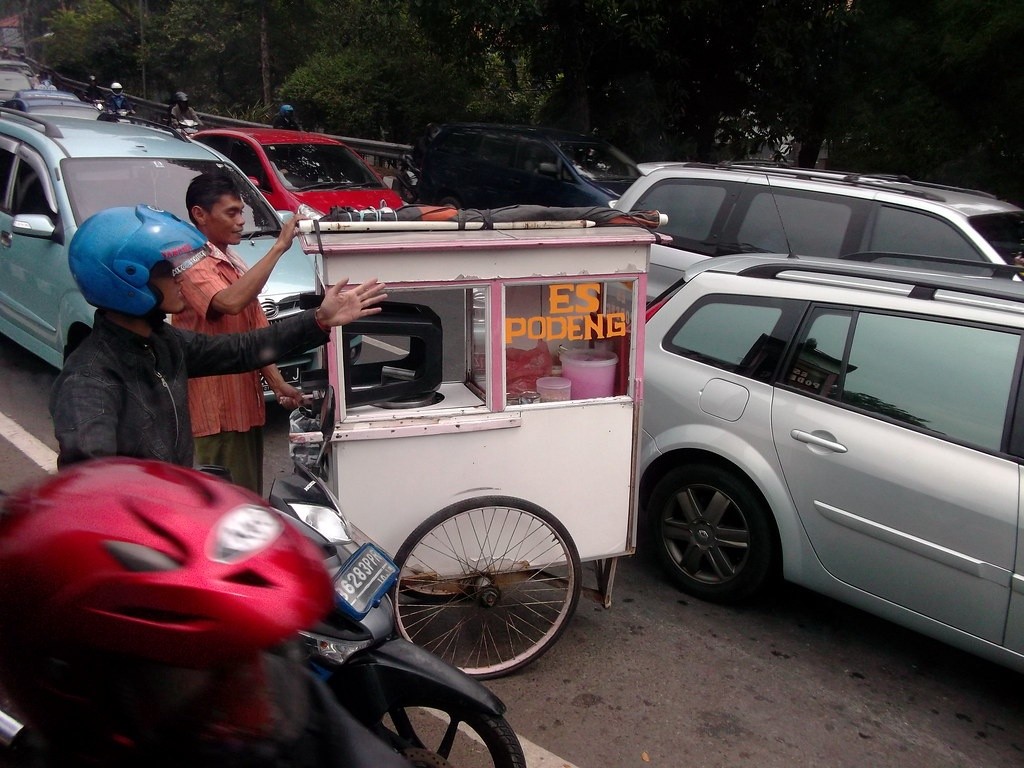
[[0, 113, 328, 414], [632, 253, 1024, 673], [608, 160, 1024, 310]]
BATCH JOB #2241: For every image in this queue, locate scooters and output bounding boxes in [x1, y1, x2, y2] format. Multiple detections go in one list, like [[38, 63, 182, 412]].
[[170, 113, 205, 138], [105, 108, 137, 119]]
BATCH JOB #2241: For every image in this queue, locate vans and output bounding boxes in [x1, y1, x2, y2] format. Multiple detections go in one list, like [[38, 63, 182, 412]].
[[416, 123, 645, 212]]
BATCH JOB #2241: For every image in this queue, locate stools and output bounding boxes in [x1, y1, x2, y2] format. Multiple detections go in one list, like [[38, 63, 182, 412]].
[[298, 293, 444, 415]]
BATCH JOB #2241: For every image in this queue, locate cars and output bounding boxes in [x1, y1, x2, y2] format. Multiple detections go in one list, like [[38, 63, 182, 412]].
[[0, 55, 104, 120], [192, 129, 405, 221]]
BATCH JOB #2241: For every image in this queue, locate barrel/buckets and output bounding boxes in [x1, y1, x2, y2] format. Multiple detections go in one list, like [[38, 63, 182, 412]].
[[559, 349, 619, 400]]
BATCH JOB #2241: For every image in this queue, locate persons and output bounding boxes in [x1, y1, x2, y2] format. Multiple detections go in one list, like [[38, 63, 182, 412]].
[[271, 103, 302, 132], [81, 74, 106, 106], [3, 464, 341, 768], [167, 164, 318, 499], [104, 83, 135, 118], [169, 92, 203, 132], [46, 200, 388, 477]]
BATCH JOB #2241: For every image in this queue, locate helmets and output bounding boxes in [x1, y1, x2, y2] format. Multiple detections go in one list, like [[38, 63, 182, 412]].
[[0, 457, 332, 768], [67, 203, 209, 317]]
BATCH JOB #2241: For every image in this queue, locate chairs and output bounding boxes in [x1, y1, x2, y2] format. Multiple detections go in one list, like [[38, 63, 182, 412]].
[[14, 171, 55, 221]]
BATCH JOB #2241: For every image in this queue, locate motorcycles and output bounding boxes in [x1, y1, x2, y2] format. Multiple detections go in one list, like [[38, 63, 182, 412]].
[[203, 456, 528, 768]]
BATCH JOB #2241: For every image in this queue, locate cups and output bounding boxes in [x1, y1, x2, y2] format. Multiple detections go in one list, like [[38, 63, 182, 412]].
[[519, 391, 540, 405], [536, 377, 571, 402]]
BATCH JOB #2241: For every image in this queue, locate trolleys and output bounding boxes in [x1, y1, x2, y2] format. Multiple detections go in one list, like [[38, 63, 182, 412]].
[[281, 200, 674, 679]]
